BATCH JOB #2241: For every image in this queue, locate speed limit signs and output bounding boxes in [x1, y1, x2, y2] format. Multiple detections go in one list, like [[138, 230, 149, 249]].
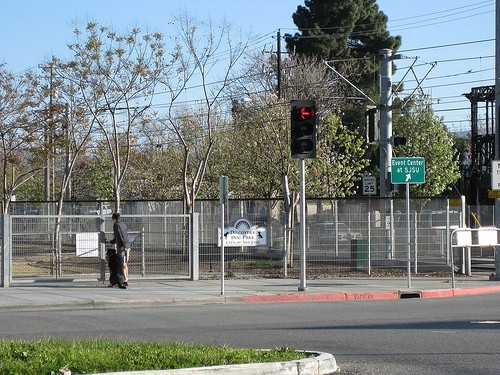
[[362, 176, 377, 196]]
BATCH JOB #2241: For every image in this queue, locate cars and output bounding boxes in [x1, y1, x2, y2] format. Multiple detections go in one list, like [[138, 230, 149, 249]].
[[308, 222, 366, 250]]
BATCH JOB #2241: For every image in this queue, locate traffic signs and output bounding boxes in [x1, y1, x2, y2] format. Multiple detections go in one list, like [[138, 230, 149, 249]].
[[391, 157, 425, 184]]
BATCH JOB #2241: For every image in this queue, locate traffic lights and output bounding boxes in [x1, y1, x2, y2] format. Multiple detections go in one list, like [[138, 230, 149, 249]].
[[291, 99, 318, 159]]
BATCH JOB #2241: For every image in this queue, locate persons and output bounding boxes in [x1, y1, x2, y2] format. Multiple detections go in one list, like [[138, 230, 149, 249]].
[[106, 212, 131, 285]]
